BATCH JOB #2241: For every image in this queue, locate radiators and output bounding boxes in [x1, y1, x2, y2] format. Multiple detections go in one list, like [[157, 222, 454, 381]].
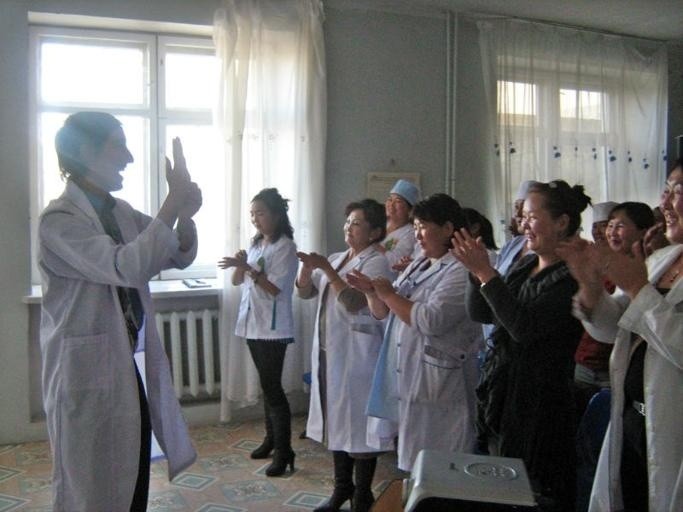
[[155, 309, 220, 398]]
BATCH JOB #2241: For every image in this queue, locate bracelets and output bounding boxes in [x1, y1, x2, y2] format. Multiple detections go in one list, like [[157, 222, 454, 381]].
[[253, 272, 261, 284], [329, 274, 340, 286]]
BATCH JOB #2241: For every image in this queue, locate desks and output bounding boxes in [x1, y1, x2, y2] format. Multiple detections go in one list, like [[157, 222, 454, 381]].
[[369, 479, 402, 512]]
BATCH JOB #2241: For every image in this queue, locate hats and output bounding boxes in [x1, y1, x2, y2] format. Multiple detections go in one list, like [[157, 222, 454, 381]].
[[592, 203, 617, 224], [514, 180, 537, 199], [392, 180, 420, 208]]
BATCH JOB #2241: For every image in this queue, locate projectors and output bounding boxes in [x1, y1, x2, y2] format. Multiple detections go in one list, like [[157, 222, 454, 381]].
[[401, 449, 538, 512]]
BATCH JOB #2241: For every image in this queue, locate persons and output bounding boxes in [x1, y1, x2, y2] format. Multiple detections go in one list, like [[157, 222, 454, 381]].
[[294, 199, 388, 511], [348, 159, 682, 512], [37, 111, 202, 511], [217, 189, 298, 476]]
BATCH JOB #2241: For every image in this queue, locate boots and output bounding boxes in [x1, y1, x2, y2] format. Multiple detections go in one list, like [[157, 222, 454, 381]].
[[313, 450, 355, 511], [350, 458, 375, 512], [252, 398, 295, 476]]
